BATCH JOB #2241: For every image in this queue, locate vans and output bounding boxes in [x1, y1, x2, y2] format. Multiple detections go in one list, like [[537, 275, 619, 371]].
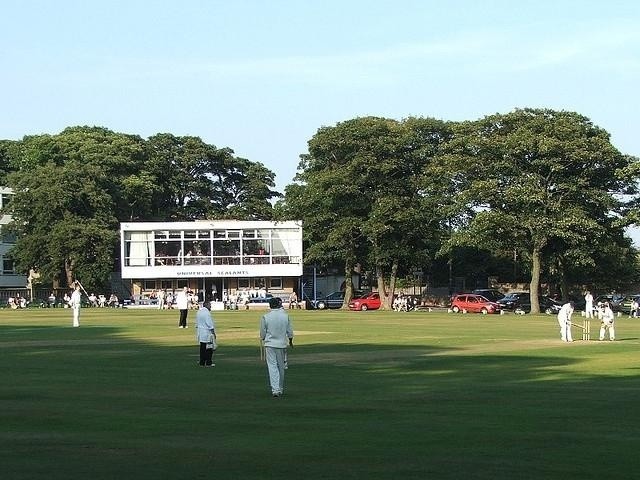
[[473, 289, 505, 301]]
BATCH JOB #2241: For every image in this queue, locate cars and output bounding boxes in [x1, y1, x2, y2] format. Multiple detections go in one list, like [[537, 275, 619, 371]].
[[450, 294, 498, 314], [496, 293, 529, 312], [349, 292, 395, 311], [574, 295, 640, 315], [520, 296, 564, 314]]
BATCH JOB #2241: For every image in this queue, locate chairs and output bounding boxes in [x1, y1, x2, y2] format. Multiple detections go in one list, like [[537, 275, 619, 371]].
[[8, 294, 120, 308], [156, 254, 290, 264]]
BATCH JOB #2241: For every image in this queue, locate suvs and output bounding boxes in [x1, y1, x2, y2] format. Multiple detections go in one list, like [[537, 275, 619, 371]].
[[312, 291, 364, 310]]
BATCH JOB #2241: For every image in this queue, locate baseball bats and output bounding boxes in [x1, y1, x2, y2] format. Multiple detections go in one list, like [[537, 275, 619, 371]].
[[570, 323, 584, 328]]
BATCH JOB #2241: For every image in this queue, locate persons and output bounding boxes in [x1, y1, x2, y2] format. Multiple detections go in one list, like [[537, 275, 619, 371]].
[[186, 288, 300, 310], [150, 288, 173, 310], [597, 304, 615, 340], [557, 301, 576, 340], [584, 292, 595, 318], [260, 298, 294, 396], [629, 301, 638, 319], [8, 294, 28, 308], [197, 301, 219, 367], [393, 293, 419, 311], [70, 286, 82, 328], [49, 292, 119, 308], [155, 248, 267, 265], [177, 286, 190, 328]]
